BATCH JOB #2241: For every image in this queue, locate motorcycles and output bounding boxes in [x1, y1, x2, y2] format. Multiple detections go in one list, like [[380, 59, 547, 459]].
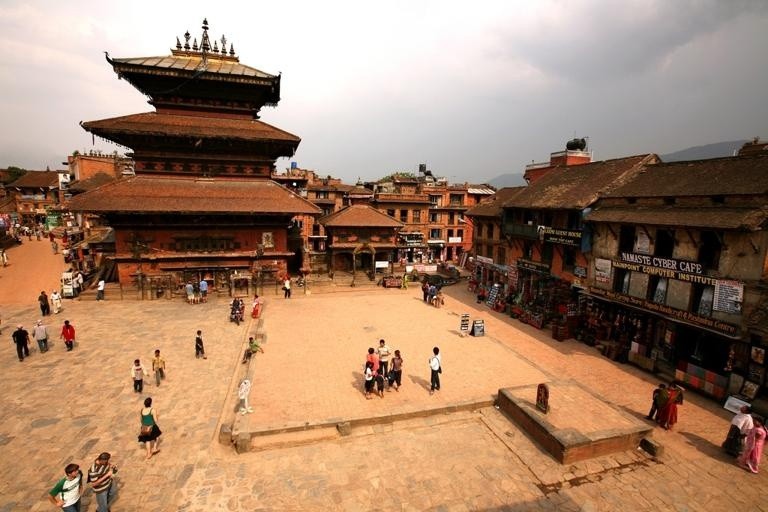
[[229, 308, 245, 326]]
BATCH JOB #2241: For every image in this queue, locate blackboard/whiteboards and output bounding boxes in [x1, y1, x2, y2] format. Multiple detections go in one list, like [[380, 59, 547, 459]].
[[473, 320, 483, 337], [488, 285, 499, 305]]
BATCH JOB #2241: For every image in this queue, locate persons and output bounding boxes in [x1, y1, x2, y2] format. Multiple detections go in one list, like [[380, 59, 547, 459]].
[[720, 406, 767, 474], [156, 283, 165, 298], [428, 346, 441, 395], [476, 286, 525, 319], [140, 397, 162, 459], [643, 381, 683, 431], [231, 293, 260, 322], [278, 273, 291, 299], [400, 268, 419, 290], [364, 339, 404, 398], [48, 453, 118, 511], [368, 271, 375, 281], [1, 221, 105, 361], [185, 278, 208, 305], [195, 330, 207, 359], [131, 348, 166, 393], [421, 280, 444, 308], [241, 337, 264, 364], [442, 259, 448, 273]]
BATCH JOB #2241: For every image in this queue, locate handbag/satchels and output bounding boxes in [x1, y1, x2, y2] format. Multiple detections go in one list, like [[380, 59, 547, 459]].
[[438, 366, 442, 374], [140, 425, 152, 435]]
